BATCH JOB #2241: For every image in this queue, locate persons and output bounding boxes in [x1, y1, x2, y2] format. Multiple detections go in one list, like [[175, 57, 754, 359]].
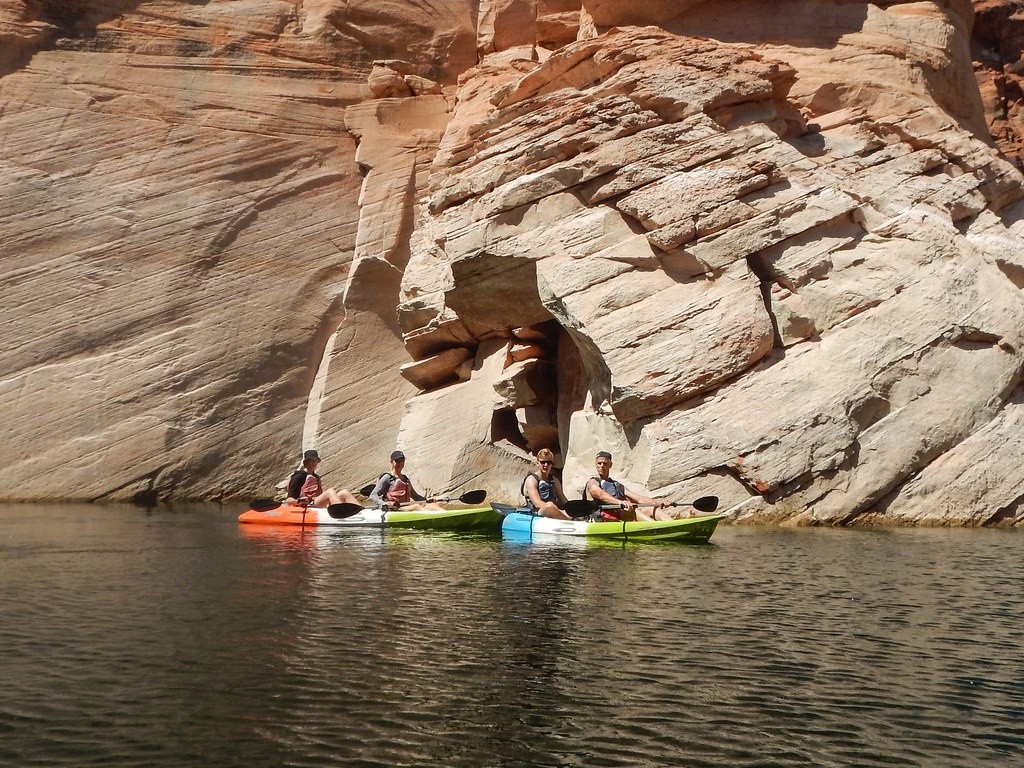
[[370, 451, 446, 511], [286, 450, 360, 508], [582, 451, 695, 521], [521, 449, 590, 520]]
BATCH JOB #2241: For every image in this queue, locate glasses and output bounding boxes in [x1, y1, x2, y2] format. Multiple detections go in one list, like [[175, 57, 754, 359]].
[[539, 460, 552, 464]]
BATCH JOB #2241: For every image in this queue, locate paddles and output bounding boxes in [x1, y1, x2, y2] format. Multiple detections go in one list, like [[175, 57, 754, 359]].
[[564, 496, 719, 519], [248, 483, 377, 513], [326, 489, 488, 519], [489, 501, 566, 515]]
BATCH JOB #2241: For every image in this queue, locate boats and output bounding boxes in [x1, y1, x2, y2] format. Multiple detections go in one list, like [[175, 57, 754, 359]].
[[502, 503, 729, 544], [238, 502, 502, 528]]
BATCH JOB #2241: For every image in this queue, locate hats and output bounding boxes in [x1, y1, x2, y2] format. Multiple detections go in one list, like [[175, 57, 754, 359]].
[[596, 451, 611, 461], [302, 450, 321, 464], [391, 451, 405, 459]]
